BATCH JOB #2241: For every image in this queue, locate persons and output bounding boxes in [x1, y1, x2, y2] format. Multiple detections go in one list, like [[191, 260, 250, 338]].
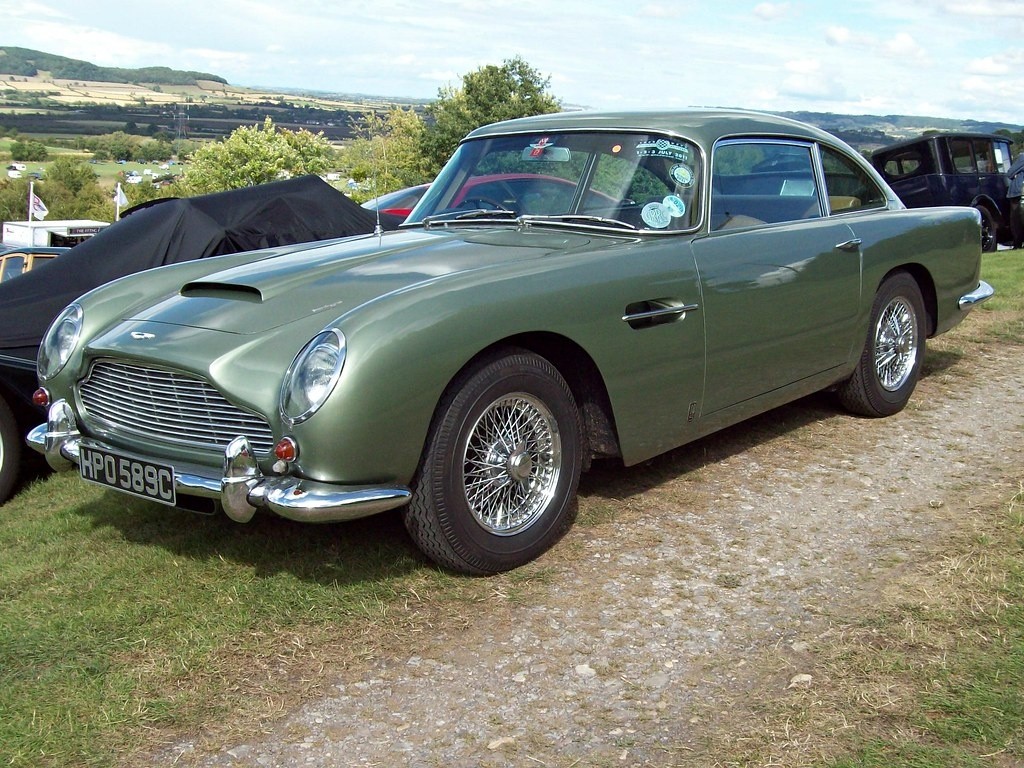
[[1005, 153, 1024, 250]]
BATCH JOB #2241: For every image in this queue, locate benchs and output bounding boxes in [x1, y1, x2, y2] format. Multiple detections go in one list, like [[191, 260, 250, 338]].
[[644, 195, 861, 223]]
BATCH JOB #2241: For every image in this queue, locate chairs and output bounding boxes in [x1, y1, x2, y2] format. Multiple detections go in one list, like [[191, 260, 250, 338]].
[[711, 214, 767, 231]]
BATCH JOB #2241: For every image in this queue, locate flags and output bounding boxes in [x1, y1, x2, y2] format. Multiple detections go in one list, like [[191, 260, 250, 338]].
[[112, 186, 129, 207], [30, 192, 50, 221]]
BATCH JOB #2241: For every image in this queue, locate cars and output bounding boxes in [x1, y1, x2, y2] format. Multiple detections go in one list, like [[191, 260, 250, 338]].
[[27, 107, 994, 577], [359, 174, 619, 219], [0, 247, 71, 284], [852, 129, 1024, 252]]
